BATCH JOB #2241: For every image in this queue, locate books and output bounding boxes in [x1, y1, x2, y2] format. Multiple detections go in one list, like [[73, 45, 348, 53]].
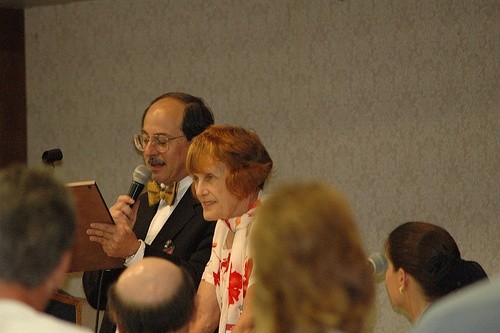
[[64, 180, 118, 273]]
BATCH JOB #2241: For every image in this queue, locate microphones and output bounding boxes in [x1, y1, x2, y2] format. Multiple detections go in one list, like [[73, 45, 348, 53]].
[[125, 165, 152, 209], [367, 253, 388, 276]]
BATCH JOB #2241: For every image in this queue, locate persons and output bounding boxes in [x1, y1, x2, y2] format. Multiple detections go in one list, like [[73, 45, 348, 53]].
[[382, 221, 488, 326], [108, 257, 195, 332], [1, 166, 94, 333], [247, 179, 374, 333], [185, 124, 273, 333], [82, 93, 218, 333]]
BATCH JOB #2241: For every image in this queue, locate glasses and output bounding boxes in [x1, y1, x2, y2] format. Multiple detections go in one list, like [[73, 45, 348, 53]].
[[133, 132, 184, 153]]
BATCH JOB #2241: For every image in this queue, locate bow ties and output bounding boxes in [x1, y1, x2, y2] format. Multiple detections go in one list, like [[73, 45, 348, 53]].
[[147, 180, 179, 207]]
